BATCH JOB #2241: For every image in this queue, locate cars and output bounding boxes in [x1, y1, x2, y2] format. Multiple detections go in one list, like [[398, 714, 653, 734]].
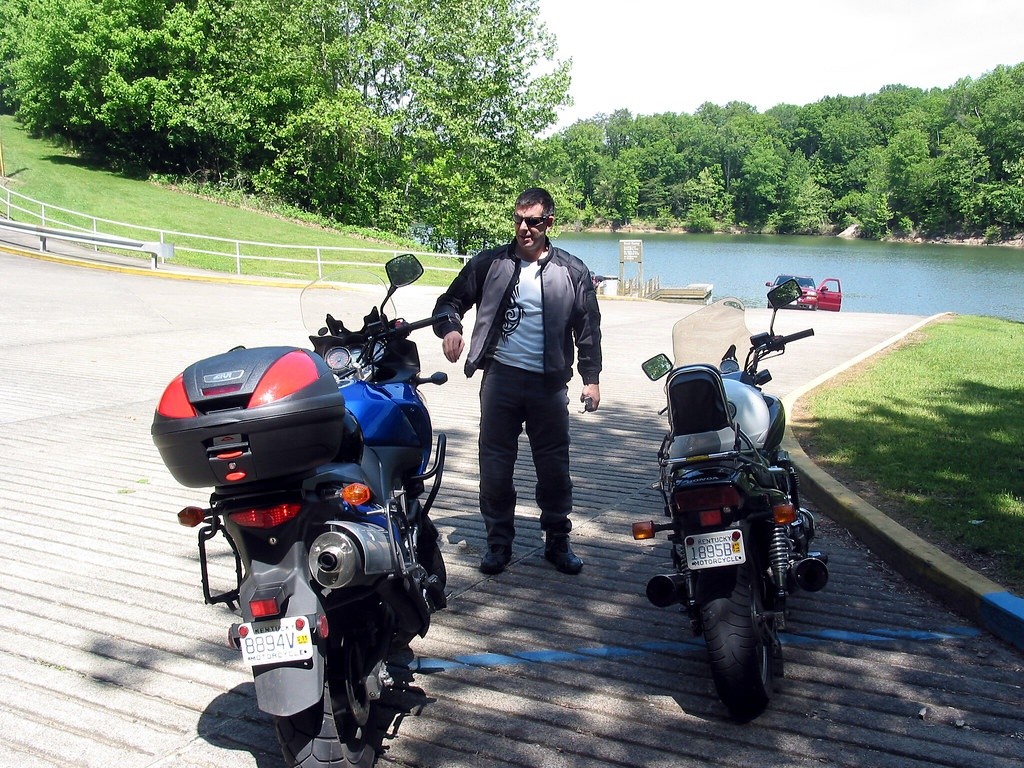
[[766, 274, 843, 313]]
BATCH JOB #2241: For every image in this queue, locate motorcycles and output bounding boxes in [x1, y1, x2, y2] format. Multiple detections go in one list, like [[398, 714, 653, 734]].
[[149, 254, 459, 765], [628, 279, 831, 714]]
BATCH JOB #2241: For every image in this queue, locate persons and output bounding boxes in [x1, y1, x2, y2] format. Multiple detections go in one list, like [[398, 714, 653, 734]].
[[432, 189, 602, 575]]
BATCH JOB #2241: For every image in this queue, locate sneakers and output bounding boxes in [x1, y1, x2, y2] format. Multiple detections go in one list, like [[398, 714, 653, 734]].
[[480, 544, 512, 574], [545, 535, 584, 574]]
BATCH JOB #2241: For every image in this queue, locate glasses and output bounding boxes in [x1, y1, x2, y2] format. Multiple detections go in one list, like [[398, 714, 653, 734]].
[[511, 212, 548, 226]]
